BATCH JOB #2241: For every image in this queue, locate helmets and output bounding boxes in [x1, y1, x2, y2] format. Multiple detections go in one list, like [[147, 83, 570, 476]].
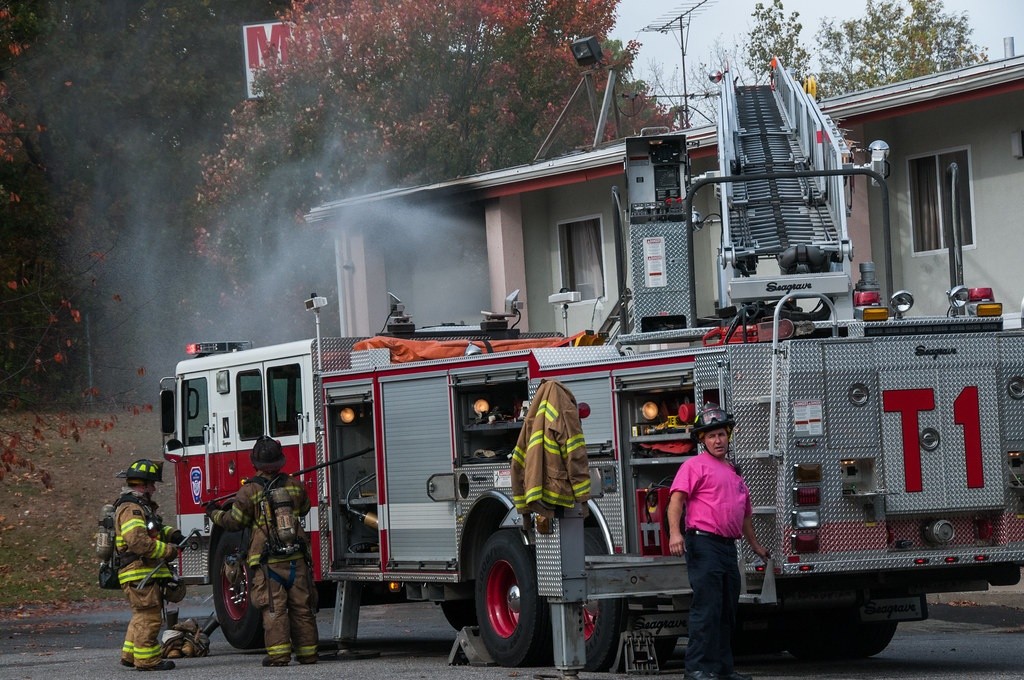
[[161, 579, 186, 603], [250, 435, 285, 472], [690, 402, 735, 444], [126, 459, 164, 483]]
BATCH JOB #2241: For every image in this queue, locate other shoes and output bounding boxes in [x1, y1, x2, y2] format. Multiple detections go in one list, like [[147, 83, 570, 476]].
[[262, 653, 291, 666], [121, 659, 134, 667], [719, 672, 752, 680], [684, 669, 720, 680], [296, 653, 319, 664], [136, 660, 175, 671]]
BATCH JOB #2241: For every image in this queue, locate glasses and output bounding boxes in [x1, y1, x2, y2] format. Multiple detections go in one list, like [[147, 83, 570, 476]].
[[695, 409, 728, 425]]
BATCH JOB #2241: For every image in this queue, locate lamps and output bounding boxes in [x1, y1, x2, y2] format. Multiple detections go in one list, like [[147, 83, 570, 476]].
[[566, 36, 616, 69], [383, 291, 416, 331], [546, 289, 582, 339], [303, 293, 327, 372], [481, 289, 523, 330]]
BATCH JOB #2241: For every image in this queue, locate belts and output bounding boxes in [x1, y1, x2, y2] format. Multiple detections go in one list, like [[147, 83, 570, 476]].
[[685, 530, 735, 545]]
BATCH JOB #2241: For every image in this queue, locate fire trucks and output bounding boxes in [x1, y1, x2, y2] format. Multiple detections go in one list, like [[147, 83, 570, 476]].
[[159, 55, 1023, 670]]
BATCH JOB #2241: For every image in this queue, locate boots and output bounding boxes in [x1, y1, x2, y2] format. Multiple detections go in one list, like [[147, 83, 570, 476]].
[[165, 608, 220, 658]]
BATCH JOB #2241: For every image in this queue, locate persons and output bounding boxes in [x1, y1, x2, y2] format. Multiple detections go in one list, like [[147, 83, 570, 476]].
[[206, 438, 319, 666], [114, 460, 186, 671], [667, 408, 771, 680]]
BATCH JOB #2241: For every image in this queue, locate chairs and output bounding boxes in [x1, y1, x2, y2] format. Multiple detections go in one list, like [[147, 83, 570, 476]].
[[241, 389, 274, 439]]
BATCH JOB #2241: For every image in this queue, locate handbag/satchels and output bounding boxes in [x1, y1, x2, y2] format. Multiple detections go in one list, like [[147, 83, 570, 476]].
[[99, 561, 121, 589]]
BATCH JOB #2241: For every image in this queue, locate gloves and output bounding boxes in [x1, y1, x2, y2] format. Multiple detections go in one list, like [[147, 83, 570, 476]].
[[164, 543, 179, 562], [170, 531, 187, 551], [206, 501, 221, 517]]
